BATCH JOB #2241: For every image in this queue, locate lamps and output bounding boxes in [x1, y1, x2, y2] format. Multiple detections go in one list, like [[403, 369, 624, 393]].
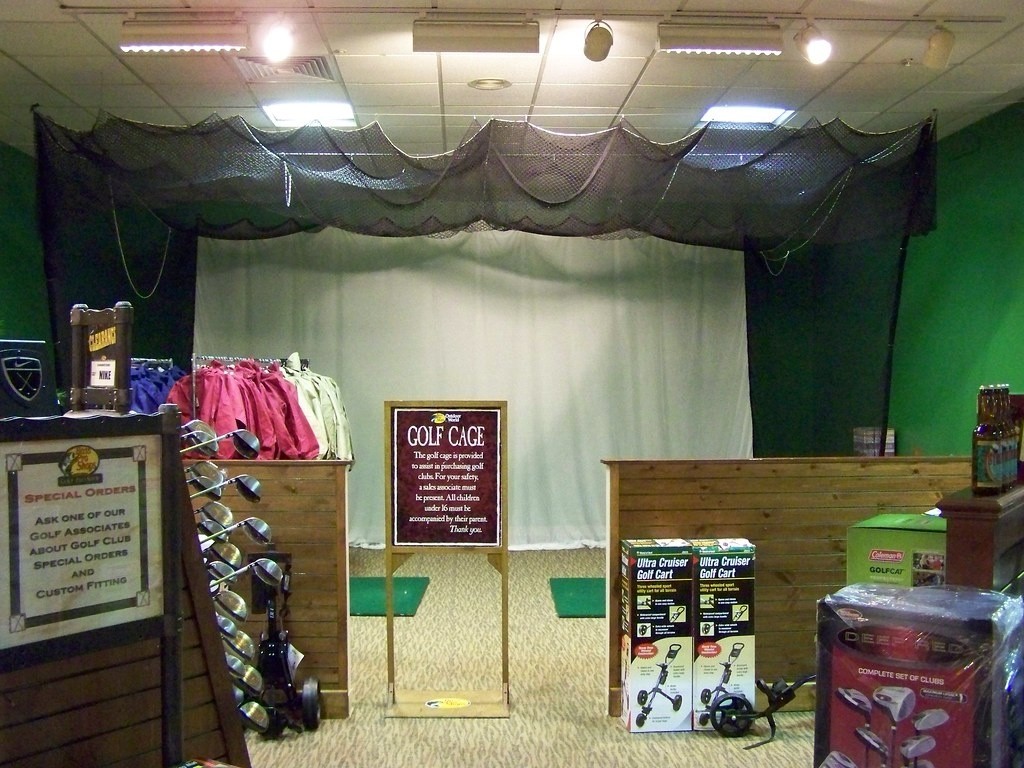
[[921, 22, 956, 70], [119, 10, 249, 52], [584, 20, 613, 62], [656, 16, 785, 56], [412, 13, 539, 53], [793, 20, 832, 65]]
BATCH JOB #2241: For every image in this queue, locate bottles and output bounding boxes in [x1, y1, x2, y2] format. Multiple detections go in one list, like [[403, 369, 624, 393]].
[[971, 384, 1024, 497]]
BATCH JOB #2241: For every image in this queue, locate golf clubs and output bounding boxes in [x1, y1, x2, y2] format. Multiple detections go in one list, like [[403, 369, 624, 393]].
[[177, 418, 288, 733], [817, 684, 951, 768]]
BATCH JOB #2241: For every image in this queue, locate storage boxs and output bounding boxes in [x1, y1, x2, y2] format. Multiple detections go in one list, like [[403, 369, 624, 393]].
[[853, 427, 895, 458], [621, 538, 755, 732], [813, 582, 1024, 768], [846, 514, 947, 585]]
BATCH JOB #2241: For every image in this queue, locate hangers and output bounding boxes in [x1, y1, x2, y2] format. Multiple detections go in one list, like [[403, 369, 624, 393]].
[[196, 356, 309, 373], [129, 358, 172, 369]]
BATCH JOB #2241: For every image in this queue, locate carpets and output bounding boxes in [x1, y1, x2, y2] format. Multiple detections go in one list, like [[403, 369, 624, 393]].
[[550, 576, 607, 619], [350, 576, 429, 618]]
